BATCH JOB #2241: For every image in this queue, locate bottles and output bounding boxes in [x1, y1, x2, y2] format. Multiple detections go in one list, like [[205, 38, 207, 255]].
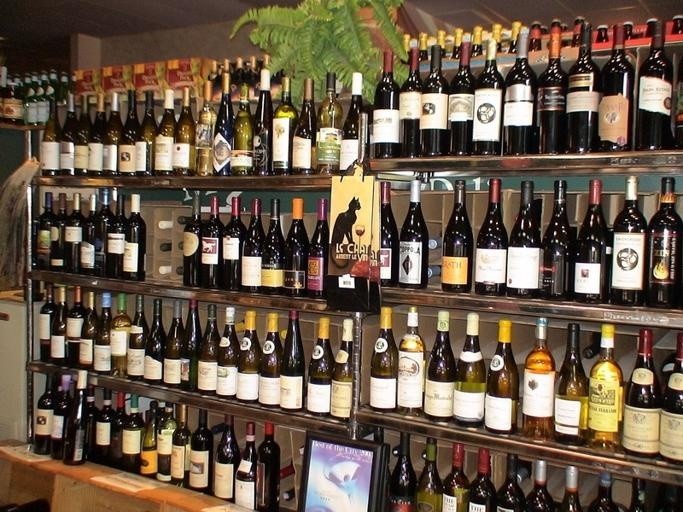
[[178, 299, 201, 391], [471, 38, 504, 157], [158, 265, 171, 275], [176, 215, 191, 226], [371, 425, 391, 485], [491, 23, 503, 52], [138, 400, 159, 476], [279, 310, 305, 411], [427, 263, 442, 280], [596, 22, 634, 154], [588, 323, 623, 449], [175, 266, 184, 276], [108, 391, 129, 466], [528, 21, 541, 52], [305, 316, 334, 415], [508, 21, 522, 54], [155, 402, 176, 482], [259, 198, 285, 294], [419, 44, 451, 157], [221, 196, 246, 291], [49, 373, 72, 459], [279, 457, 295, 479], [158, 241, 171, 253], [469, 25, 484, 57], [483, 319, 519, 433], [200, 195, 224, 290], [156, 218, 173, 231], [34, 191, 55, 269], [553, 321, 589, 445], [569, 16, 585, 47], [37, 282, 57, 362], [386, 430, 416, 512], [398, 38, 423, 158], [58, 91, 78, 174], [256, 422, 280, 511], [174, 87, 196, 176], [126, 294, 149, 380], [564, 21, 602, 154], [211, 413, 240, 504], [659, 331, 683, 379], [64, 191, 85, 274], [120, 192, 148, 281], [229, 81, 253, 178], [503, 29, 536, 155], [627, 476, 647, 511], [595, 24, 609, 43], [91, 292, 113, 377], [109, 292, 131, 378], [188, 408, 213, 492], [644, 17, 659, 38], [65, 285, 88, 365], [133, 90, 158, 176], [520, 317, 557, 442], [93, 187, 115, 280], [394, 303, 427, 416], [547, 18, 562, 51], [207, 54, 273, 80], [79, 193, 97, 276], [121, 392, 144, 471], [338, 71, 368, 172], [153, 88, 178, 176], [305, 197, 330, 299], [162, 300, 184, 388], [81, 383, 101, 460], [398, 180, 429, 291], [445, 41, 475, 158], [282, 486, 295, 501], [314, 71, 343, 176], [634, 20, 672, 152], [33, 371, 52, 456], [573, 178, 611, 303], [290, 77, 316, 174], [48, 191, 70, 270], [377, 181, 400, 287], [505, 179, 541, 298], [142, 298, 166, 385], [116, 90, 140, 178], [181, 196, 207, 287], [647, 176, 682, 310], [470, 447, 496, 511], [169, 403, 191, 482], [193, 81, 218, 177], [620, 328, 663, 456], [400, 33, 412, 65], [214, 307, 240, 398], [233, 420, 256, 510], [442, 441, 471, 511], [621, 21, 634, 42], [440, 179, 473, 295], [367, 304, 398, 411], [93, 386, 116, 462], [270, 76, 298, 175], [675, 57, 683, 152], [450, 28, 464, 59], [421, 309, 456, 420], [73, 93, 94, 175], [426, 230, 444, 250], [49, 285, 68, 364], [418, 31, 429, 60], [60, 369, 88, 465], [196, 303, 220, 395], [39, 96, 62, 177], [453, 310, 487, 425], [436, 30, 447, 57], [87, 92, 106, 178], [284, 198, 309, 297], [527, 457, 556, 510], [252, 69, 273, 175], [212, 72, 236, 177], [102, 93, 124, 178], [495, 452, 527, 511], [542, 178, 576, 302], [76, 291, 98, 368], [329, 318, 355, 421], [105, 192, 127, 280], [7, 69, 70, 96], [0, 37, 6, 95], [241, 197, 266, 293], [657, 343, 683, 465], [371, 50, 401, 159], [0, 97, 39, 125], [609, 173, 649, 307], [533, 27, 566, 155], [257, 312, 283, 407], [585, 469, 621, 512], [556, 464, 584, 512], [235, 309, 261, 403], [177, 240, 182, 251], [416, 436, 442, 510], [670, 15, 682, 36]]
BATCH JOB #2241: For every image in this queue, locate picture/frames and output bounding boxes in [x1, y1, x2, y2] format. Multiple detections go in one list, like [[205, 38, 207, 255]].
[[299, 429, 391, 512]]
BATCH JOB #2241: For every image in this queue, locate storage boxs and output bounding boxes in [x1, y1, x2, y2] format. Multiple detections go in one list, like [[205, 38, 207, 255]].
[[73, 56, 222, 105]]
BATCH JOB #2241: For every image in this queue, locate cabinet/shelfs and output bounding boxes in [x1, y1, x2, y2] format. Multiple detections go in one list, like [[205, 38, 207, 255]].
[[0, 91, 354, 512], [353, 38, 683, 511]]
